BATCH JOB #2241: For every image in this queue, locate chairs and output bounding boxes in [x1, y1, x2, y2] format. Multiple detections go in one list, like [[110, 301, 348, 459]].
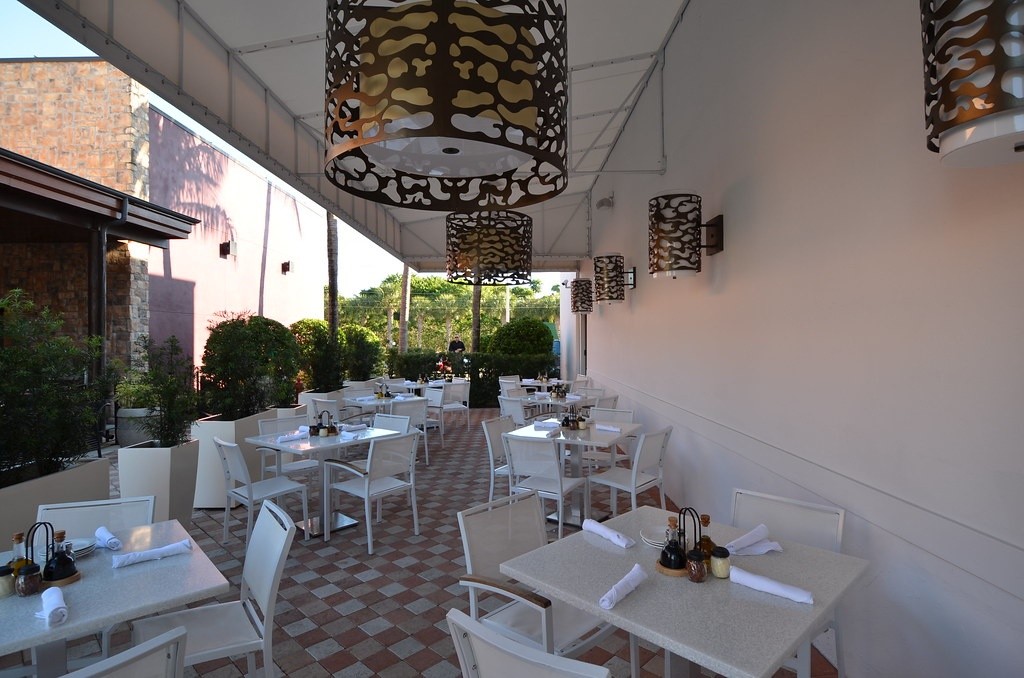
[[37, 377, 849, 678]]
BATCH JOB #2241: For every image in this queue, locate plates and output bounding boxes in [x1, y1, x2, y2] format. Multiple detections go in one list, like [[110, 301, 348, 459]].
[[640, 526, 668, 548], [38, 538, 97, 561]]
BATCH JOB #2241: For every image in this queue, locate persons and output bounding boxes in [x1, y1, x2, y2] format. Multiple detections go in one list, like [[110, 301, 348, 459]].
[[449, 335, 465, 377]]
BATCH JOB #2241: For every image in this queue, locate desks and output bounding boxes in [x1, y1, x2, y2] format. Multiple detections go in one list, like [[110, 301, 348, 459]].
[[507, 417, 644, 530], [0, 518, 230, 678], [342, 393, 432, 414], [526, 393, 595, 457], [244, 424, 401, 538], [404, 380, 451, 428], [500, 504, 869, 678], [520, 380, 570, 415]]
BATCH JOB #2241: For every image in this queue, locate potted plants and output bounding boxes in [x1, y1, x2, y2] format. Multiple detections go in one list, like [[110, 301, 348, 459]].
[[0, 285, 390, 550]]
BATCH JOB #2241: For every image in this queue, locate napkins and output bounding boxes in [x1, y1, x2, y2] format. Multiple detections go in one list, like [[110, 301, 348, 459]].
[[112, 539, 193, 569], [598, 563, 648, 610], [95, 524, 124, 550], [724, 523, 784, 556], [276, 431, 308, 442], [582, 518, 636, 549], [546, 426, 560, 438], [730, 565, 814, 605], [342, 423, 367, 431], [34, 586, 69, 627], [533, 420, 560, 427], [594, 423, 622, 433]]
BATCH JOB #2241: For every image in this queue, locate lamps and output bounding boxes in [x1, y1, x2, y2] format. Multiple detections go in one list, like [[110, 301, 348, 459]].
[[919, 0, 1024, 154], [220, 0, 725, 314]]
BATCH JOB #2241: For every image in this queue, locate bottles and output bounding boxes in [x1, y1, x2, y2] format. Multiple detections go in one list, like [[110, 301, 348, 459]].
[[319, 426, 328, 437], [417, 374, 429, 384], [576, 409, 586, 430], [374, 383, 391, 399], [317, 415, 323, 434], [570, 420, 577, 430], [328, 415, 337, 436], [562, 411, 570, 427], [694, 514, 716, 573], [0, 565, 15, 599], [15, 564, 42, 597], [64, 543, 76, 560], [309, 426, 319, 436], [550, 384, 566, 398], [43, 530, 78, 581], [661, 516, 685, 569], [686, 550, 707, 583], [537, 372, 548, 382], [711, 547, 730, 579], [6, 531, 33, 577]]
